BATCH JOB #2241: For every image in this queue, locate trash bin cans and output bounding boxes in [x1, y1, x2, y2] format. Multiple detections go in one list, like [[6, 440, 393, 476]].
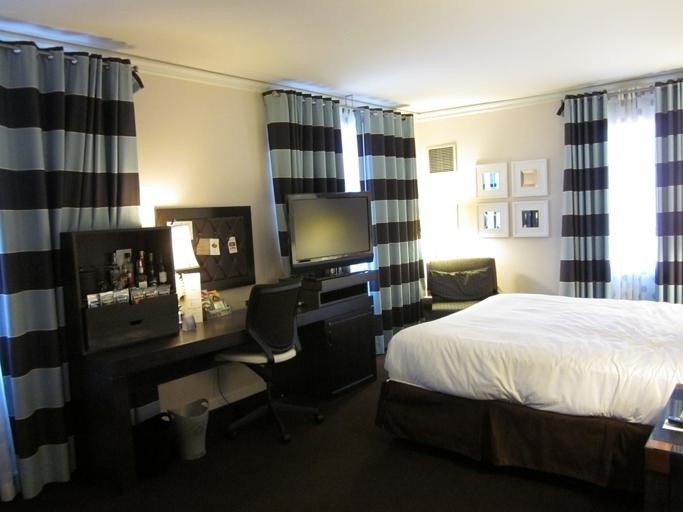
[[164, 398, 220, 462]]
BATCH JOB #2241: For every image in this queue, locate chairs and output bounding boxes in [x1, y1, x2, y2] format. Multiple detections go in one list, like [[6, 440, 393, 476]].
[[427, 258, 497, 320], [215, 282, 325, 444]]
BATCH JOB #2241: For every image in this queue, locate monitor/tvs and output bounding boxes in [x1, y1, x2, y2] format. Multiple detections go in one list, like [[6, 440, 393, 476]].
[[287, 192, 374, 280]]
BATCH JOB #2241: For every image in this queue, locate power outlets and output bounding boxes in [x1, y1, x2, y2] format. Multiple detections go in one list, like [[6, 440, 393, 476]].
[[244, 300, 249, 308]]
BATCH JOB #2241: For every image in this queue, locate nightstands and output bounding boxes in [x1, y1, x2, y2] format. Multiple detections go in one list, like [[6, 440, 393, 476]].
[[644, 384, 683, 512]]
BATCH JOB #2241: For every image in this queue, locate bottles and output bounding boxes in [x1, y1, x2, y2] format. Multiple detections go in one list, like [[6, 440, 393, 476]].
[[136, 267, 148, 288], [135, 251, 145, 274], [146, 253, 157, 287], [107, 252, 134, 288], [155, 253, 167, 284]]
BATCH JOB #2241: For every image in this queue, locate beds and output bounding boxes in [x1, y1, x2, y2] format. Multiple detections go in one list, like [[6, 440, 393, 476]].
[[375, 293, 683, 496]]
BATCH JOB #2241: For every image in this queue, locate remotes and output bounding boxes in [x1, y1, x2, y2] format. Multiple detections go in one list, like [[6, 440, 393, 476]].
[[668, 415, 682, 427]]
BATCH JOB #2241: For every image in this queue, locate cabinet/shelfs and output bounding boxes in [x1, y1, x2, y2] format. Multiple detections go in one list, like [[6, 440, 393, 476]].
[[60, 226, 180, 360]]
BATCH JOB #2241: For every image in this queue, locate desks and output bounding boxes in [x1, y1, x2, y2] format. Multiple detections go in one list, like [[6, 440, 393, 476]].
[[70, 271, 378, 490]]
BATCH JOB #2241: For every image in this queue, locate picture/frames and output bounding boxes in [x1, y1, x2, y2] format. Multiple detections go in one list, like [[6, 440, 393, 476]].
[[511, 159, 548, 198], [476, 202, 510, 238], [474, 162, 509, 201], [512, 201, 549, 238]]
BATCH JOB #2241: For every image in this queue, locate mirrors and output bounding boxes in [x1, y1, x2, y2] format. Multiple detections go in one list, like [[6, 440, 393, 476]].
[[155, 206, 255, 291]]
